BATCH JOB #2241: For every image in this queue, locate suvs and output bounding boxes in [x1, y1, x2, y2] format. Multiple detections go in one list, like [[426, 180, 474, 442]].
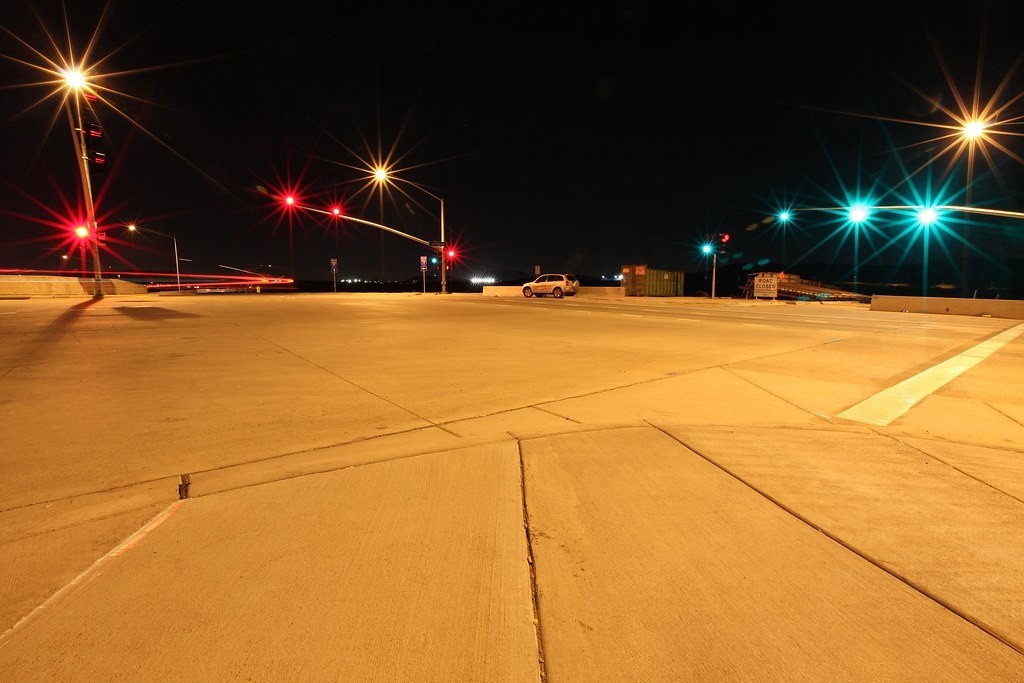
[[522, 273, 579, 298]]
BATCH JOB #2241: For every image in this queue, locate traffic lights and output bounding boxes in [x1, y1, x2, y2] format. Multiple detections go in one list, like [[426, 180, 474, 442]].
[[780, 204, 789, 223], [719, 233, 729, 252]]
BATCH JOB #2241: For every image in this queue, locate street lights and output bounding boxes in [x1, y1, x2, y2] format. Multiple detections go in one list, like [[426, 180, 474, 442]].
[[127, 225, 181, 292], [376, 169, 446, 294], [61, 70, 105, 297]]
